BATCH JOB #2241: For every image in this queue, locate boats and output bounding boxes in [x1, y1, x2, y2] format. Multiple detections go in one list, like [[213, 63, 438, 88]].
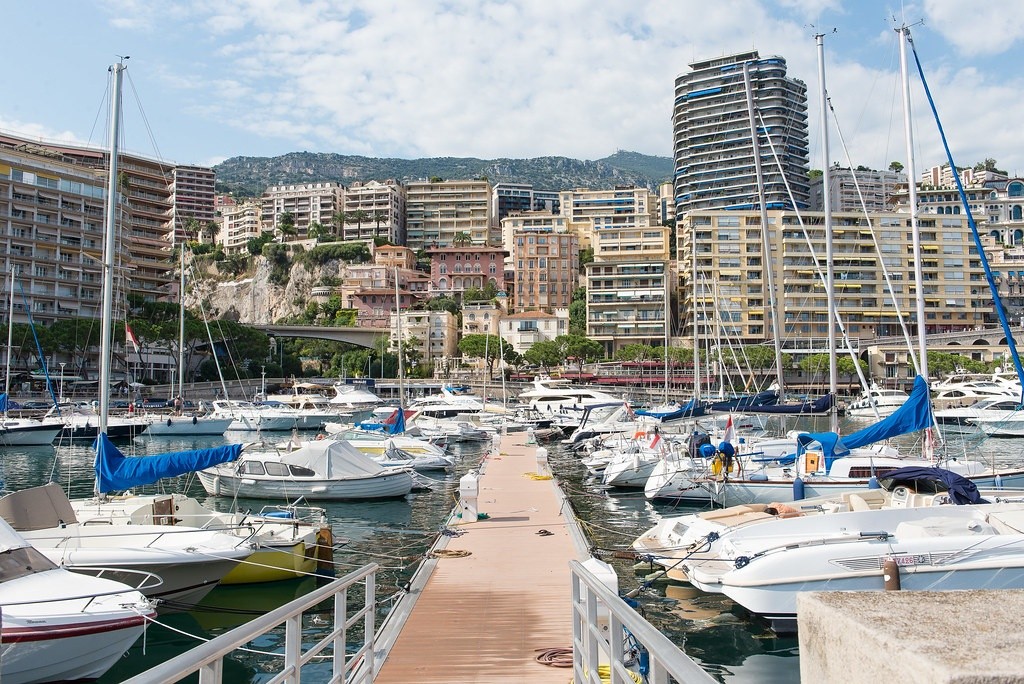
[[0, 510, 165, 684]]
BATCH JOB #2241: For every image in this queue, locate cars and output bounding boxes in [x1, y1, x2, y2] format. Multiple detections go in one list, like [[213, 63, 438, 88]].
[[5, 395, 195, 412]]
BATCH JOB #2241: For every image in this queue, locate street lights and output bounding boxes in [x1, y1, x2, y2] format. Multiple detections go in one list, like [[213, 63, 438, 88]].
[[45, 356, 51, 392], [58, 362, 67, 403], [260, 365, 267, 402], [170, 368, 176, 400], [368, 356, 372, 378]]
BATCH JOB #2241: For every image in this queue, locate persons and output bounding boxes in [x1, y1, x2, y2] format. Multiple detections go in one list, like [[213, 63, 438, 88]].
[[119, 386, 127, 399], [459, 384, 467, 393], [764, 503, 801, 518], [129, 401, 134, 418], [175, 397, 181, 417], [144, 398, 148, 403]]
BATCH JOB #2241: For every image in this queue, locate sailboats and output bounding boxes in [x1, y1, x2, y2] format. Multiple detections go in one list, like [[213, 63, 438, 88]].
[[0, 29, 1023, 639]]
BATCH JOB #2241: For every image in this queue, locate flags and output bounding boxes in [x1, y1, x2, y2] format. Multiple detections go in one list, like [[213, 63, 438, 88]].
[[650, 434, 665, 453], [723, 410, 735, 443], [125, 322, 140, 354]]
